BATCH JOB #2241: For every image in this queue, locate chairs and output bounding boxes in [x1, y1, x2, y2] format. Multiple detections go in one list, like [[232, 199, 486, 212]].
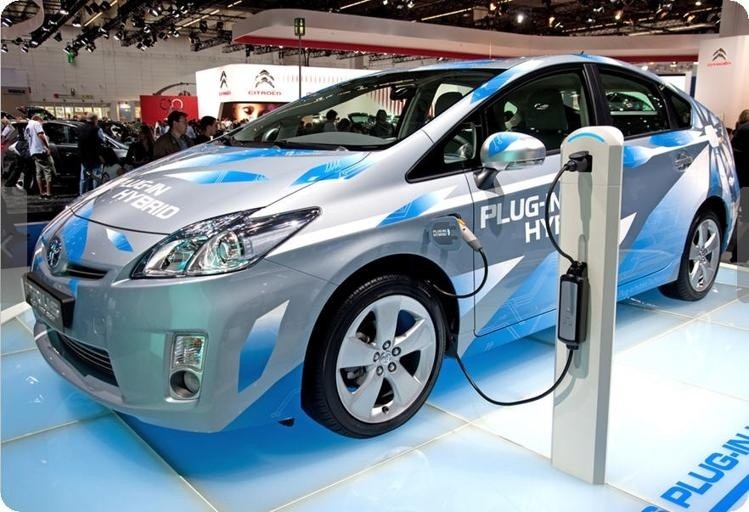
[[518, 85, 570, 150], [434, 91, 484, 160]]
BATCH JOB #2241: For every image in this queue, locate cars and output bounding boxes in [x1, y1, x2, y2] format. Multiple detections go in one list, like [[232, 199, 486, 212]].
[[22, 52, 741, 439], [1, 106, 130, 193]]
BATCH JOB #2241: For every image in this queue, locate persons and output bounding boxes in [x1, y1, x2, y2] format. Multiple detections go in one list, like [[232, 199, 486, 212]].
[[723, 108, 748, 267], [1, 111, 250, 196], [298, 103, 398, 139], [224, 103, 269, 124]]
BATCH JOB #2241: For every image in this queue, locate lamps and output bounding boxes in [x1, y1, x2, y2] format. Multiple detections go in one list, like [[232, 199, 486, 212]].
[[0, 1, 224, 59]]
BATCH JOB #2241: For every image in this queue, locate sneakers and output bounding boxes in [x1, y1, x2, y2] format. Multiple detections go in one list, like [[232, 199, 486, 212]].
[[40, 192, 58, 197]]
